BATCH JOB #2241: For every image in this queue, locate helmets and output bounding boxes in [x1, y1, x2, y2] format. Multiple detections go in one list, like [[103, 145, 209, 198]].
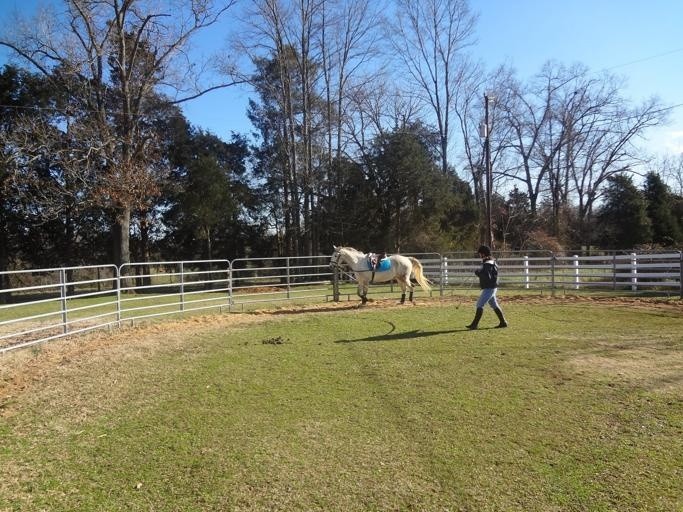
[[478, 246, 491, 252]]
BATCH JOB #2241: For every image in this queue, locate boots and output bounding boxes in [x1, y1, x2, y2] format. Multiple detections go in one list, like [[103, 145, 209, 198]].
[[465, 308, 484, 329], [494, 307, 508, 328]]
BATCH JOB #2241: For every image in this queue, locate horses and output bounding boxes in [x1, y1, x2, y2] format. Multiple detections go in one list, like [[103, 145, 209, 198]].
[[329, 244, 432, 305]]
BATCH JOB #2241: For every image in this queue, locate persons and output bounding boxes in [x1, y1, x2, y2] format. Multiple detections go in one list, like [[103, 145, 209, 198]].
[[466, 246, 507, 330]]
